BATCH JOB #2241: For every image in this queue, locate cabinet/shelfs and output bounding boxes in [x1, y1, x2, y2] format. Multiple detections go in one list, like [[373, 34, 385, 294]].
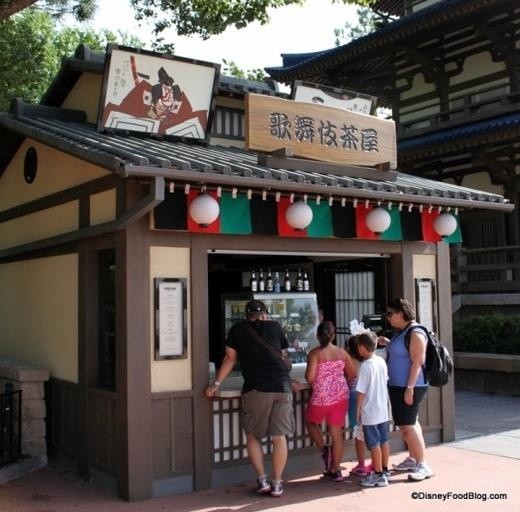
[[220, 293, 322, 370]]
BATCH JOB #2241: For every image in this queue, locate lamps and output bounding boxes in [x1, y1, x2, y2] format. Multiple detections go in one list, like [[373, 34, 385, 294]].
[[433, 209, 459, 240], [188, 190, 222, 229], [282, 197, 316, 233]]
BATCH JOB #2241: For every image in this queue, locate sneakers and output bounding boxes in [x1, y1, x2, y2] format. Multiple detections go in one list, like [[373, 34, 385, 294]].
[[350, 464, 368, 476], [391, 456, 417, 471], [384, 471, 391, 481], [359, 470, 388, 487], [254, 475, 272, 494], [323, 468, 344, 482], [363, 463, 373, 472], [406, 461, 433, 482], [271, 482, 284, 497], [322, 445, 329, 470]]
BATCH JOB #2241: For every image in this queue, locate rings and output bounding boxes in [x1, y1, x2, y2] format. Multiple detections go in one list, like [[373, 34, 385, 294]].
[[209, 392, 211, 393]]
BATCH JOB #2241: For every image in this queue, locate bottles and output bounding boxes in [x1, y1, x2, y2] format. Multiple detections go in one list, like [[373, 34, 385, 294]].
[[249, 269, 310, 292], [209, 361, 216, 386]]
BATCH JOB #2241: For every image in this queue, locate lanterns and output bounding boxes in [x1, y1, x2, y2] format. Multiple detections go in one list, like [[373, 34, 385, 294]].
[[285, 198, 313, 231], [434, 213, 457, 238], [189, 192, 220, 227]]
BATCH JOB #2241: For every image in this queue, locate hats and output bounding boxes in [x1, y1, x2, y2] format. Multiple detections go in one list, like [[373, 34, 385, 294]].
[[245, 300, 270, 316]]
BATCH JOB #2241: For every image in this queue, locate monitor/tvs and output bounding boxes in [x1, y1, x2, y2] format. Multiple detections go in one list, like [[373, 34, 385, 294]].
[[361, 313, 384, 332]]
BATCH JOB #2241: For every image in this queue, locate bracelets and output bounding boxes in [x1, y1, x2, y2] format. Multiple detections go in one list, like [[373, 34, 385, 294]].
[[406, 386, 414, 388], [213, 381, 220, 386]]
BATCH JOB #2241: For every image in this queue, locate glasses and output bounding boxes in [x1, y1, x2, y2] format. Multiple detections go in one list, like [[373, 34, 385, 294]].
[[383, 310, 400, 318]]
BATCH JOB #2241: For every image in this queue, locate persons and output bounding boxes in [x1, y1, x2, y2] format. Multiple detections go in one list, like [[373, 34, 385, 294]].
[[205, 300, 292, 497], [377, 298, 432, 481], [345, 336, 376, 476], [305, 321, 355, 482], [355, 332, 392, 487]]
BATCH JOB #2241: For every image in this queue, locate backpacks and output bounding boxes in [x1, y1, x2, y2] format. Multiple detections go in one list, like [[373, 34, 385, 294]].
[[403, 324, 454, 387]]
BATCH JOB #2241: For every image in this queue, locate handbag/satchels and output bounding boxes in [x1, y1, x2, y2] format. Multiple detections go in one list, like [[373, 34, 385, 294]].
[[276, 348, 293, 371]]
